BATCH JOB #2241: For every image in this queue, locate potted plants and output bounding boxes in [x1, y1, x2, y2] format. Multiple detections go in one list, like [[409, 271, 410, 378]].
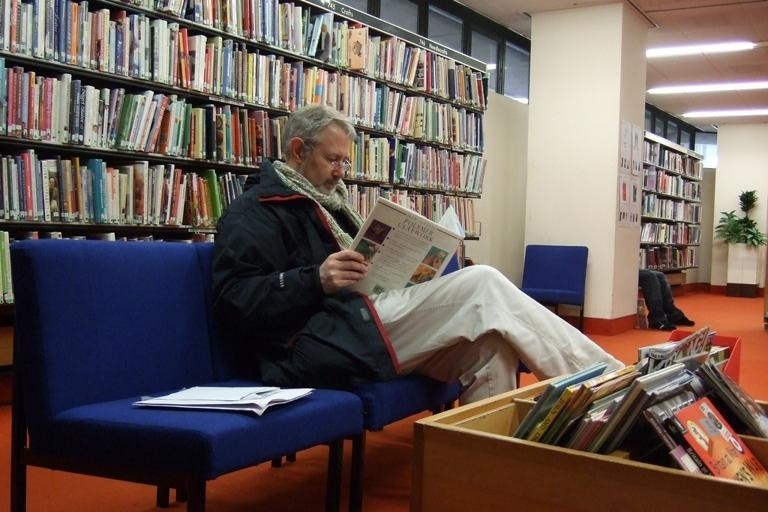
[[716, 189, 767, 299]]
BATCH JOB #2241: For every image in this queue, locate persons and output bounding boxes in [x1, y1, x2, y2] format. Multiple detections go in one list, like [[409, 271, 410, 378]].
[[213, 106, 628, 403], [640, 267, 696, 331]]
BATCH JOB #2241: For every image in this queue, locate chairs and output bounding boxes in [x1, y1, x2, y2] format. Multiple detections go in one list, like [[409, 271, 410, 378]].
[[8, 241, 362, 508], [517, 245, 590, 335], [201, 243, 480, 512]]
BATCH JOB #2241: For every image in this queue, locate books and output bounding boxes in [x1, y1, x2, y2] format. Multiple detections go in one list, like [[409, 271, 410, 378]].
[[339, 198, 463, 296], [638, 137, 703, 274], [0, 0, 487, 236], [510, 325, 768, 489], [1, 231, 14, 304], [10, 232, 214, 243]]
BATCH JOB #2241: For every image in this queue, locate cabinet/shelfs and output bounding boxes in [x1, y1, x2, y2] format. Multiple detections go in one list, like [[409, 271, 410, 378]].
[[1, 0, 496, 305], [640, 131, 702, 272]]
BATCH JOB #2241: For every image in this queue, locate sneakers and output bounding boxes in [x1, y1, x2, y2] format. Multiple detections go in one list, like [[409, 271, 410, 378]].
[[647, 314, 695, 332]]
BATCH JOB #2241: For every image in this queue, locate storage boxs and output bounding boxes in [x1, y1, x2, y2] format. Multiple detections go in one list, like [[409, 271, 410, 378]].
[[667, 328, 742, 386], [411, 370, 768, 512]]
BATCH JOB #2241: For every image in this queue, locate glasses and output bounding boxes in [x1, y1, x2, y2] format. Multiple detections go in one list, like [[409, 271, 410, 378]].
[[304, 141, 353, 172]]
